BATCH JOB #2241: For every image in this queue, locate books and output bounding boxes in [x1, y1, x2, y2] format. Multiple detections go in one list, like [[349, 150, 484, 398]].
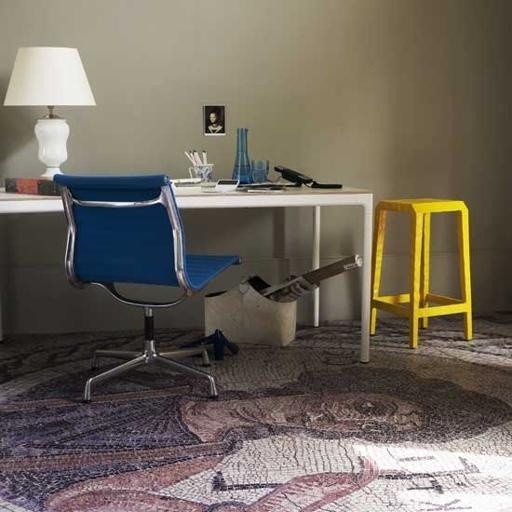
[[4, 177, 61, 196]]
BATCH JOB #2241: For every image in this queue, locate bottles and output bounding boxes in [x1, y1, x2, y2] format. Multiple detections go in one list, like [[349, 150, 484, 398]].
[[231, 127, 254, 185]]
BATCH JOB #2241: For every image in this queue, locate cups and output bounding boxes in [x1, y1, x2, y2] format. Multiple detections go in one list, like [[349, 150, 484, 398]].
[[188, 163, 215, 185], [251, 159, 270, 186]]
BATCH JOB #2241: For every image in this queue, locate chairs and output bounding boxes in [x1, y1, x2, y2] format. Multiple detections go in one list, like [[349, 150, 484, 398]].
[[54, 174, 242, 403]]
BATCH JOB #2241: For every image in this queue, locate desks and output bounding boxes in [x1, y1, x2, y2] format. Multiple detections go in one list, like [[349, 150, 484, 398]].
[[0, 182, 374, 363]]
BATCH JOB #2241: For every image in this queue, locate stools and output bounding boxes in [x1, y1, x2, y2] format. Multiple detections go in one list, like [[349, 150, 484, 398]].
[[370, 198, 472, 349]]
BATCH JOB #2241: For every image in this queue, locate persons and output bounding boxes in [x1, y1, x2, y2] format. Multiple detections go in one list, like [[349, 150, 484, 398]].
[[208, 112, 224, 133]]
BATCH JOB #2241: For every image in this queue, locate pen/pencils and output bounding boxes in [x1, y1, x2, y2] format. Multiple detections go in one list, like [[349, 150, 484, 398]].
[[247, 189, 285, 193], [185, 150, 207, 164]]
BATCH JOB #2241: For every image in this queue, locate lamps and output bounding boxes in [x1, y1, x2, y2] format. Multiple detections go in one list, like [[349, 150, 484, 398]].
[[2, 47, 96, 179]]
